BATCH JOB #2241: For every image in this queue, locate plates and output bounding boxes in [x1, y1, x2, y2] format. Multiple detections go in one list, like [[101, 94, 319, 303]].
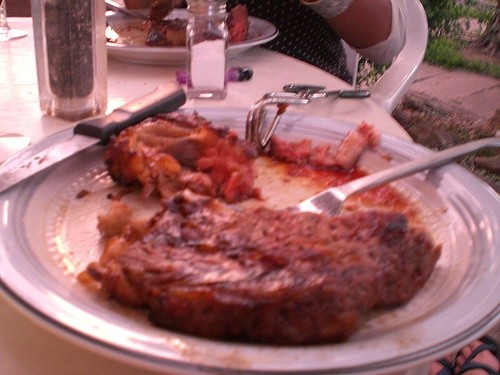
[[1, 106, 499, 375], [103, 8, 279, 65]]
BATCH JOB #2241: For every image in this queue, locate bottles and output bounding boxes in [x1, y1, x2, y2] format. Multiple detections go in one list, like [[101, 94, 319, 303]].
[[0, 0, 9, 34], [30, 1, 106, 120]]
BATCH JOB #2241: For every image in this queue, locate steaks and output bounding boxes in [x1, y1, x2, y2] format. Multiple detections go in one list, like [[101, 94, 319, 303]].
[[104, 111, 258, 199], [104, 188, 443, 344], [145, 4, 248, 47]]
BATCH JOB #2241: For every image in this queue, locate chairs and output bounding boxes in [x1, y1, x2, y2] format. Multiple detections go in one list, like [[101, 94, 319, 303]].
[[340, 0, 428, 116]]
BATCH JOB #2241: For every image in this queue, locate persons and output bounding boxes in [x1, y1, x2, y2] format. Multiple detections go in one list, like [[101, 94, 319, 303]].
[[123, 0, 408, 87]]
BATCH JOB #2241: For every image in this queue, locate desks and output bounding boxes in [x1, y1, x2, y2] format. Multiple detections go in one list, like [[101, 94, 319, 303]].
[[0, 16, 415, 375]]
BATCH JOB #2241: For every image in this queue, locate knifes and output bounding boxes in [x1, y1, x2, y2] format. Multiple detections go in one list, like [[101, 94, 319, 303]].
[[1, 80, 187, 197]]
